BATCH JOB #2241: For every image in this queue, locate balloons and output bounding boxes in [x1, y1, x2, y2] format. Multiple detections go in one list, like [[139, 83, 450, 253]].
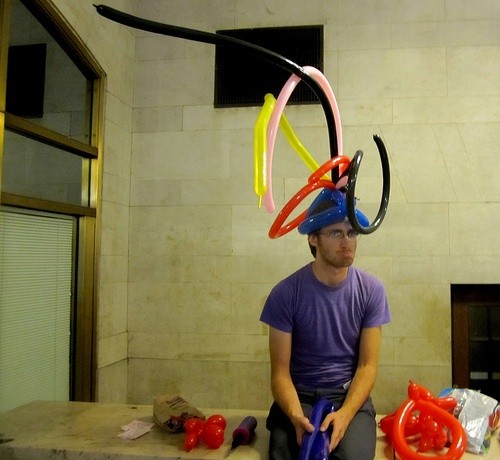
[[378, 379, 466, 460], [92, 3, 390, 240], [182, 414, 227, 452], [301, 398, 335, 460]]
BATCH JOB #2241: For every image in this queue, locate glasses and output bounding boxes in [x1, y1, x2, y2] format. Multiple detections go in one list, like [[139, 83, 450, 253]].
[[312, 230, 363, 240]]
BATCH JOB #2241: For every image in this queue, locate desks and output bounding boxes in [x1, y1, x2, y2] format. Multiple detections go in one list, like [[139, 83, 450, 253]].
[[0, 399, 500, 460]]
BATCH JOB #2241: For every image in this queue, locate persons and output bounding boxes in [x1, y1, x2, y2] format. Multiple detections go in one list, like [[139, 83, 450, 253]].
[[259, 201, 391, 460]]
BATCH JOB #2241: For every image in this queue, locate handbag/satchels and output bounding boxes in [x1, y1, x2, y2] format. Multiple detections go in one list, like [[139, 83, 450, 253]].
[[443, 384, 499, 454], [151, 393, 207, 434]]
